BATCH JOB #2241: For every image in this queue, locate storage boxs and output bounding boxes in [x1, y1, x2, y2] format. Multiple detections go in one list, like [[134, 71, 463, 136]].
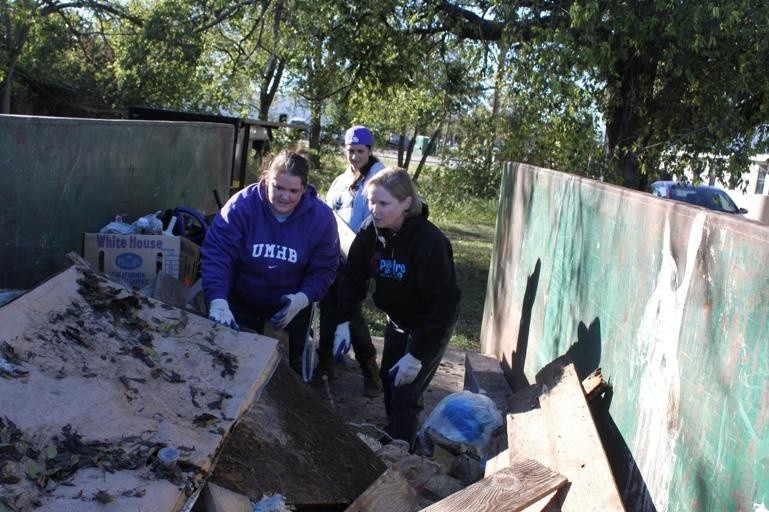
[[81, 231, 209, 315]]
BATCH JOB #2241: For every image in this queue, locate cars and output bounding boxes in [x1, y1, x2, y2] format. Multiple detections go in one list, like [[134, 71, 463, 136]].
[[651, 181, 748, 218]]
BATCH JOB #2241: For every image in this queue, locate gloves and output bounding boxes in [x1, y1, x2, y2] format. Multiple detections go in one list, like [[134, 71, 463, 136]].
[[387, 353, 422, 388], [271, 293, 309, 330], [332, 321, 351, 363], [208, 298, 240, 330]]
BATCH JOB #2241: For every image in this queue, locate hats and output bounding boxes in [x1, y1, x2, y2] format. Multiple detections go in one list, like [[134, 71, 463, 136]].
[[345, 125, 373, 146]]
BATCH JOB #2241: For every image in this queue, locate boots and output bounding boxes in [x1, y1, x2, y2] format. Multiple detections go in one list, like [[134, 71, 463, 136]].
[[357, 355, 382, 397], [310, 348, 338, 385]]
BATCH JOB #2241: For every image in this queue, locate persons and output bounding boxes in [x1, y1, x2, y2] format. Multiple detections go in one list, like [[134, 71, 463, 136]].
[[330, 164, 464, 455], [200, 149, 344, 374], [309, 120, 383, 399]]
